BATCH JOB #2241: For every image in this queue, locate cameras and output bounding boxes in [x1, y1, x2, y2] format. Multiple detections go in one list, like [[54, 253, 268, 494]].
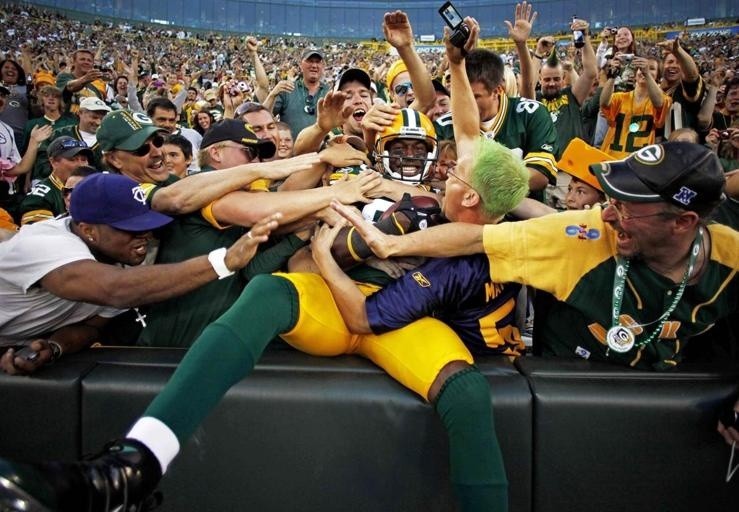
[[617, 52, 634, 68], [608, 25, 618, 36], [439, 1, 472, 47], [718, 129, 731, 141], [93, 64, 108, 79]]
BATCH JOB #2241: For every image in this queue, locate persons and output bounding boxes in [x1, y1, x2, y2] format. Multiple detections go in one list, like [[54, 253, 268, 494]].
[[418, 49, 449, 79], [128, 59, 192, 125], [56, 50, 106, 115], [194, 110, 215, 136], [308, 16, 529, 360], [21, 135, 93, 226], [307, 38, 400, 68], [704, 127, 739, 200], [509, 172, 607, 219], [0, 123, 53, 183], [594, 27, 636, 148], [56, 0, 107, 49], [599, 52, 673, 159], [0, 0, 56, 72], [0, 106, 510, 512], [270, 33, 307, 86], [192, 0, 238, 89], [263, 47, 331, 141], [146, 97, 176, 134], [501, 47, 521, 74], [292, 68, 374, 156], [697, 67, 739, 127], [234, 102, 278, 160], [62, 166, 96, 219], [537, 19, 597, 160], [331, 142, 739, 451], [179, 87, 200, 129], [137, 118, 382, 348], [532, 35, 579, 93], [237, 36, 268, 102], [28, 72, 54, 119], [425, 79, 450, 124], [433, 49, 558, 202], [107, 0, 192, 76], [0, 86, 21, 164], [204, 89, 224, 122], [636, 17, 739, 67], [276, 121, 293, 157], [0, 174, 281, 377], [501, 1, 537, 99], [112, 76, 129, 109], [23, 87, 78, 193], [656, 37, 706, 147], [0, 59, 30, 152], [161, 134, 193, 179], [97, 108, 320, 214], [385, 59, 417, 107], [428, 140, 458, 181], [49, 97, 112, 158]]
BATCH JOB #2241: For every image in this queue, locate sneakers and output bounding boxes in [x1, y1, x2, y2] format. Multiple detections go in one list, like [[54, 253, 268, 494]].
[[2, 440, 164, 512]]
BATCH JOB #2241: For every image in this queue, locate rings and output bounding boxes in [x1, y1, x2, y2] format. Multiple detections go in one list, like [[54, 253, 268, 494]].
[[247, 231, 252, 238]]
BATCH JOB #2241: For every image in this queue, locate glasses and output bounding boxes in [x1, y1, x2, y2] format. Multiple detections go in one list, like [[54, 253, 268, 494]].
[[540, 71, 564, 83], [60, 188, 74, 198], [445, 164, 475, 190], [392, 81, 414, 96], [235, 103, 266, 120], [50, 140, 88, 159], [124, 135, 165, 158], [304, 94, 314, 115], [218, 143, 261, 161], [604, 193, 680, 220]]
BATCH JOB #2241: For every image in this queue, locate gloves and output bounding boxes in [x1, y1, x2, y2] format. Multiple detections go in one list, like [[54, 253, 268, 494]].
[[395, 192, 440, 232]]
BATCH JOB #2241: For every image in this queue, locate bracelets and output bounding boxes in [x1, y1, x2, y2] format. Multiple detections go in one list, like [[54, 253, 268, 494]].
[[207, 247, 234, 279], [534, 54, 544, 60]]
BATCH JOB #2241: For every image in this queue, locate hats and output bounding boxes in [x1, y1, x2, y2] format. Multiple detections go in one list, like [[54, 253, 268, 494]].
[[47, 136, 93, 159], [387, 60, 410, 92], [556, 137, 617, 192], [141, 70, 149, 76], [200, 119, 276, 160], [204, 90, 215, 101], [431, 75, 449, 95], [333, 67, 370, 93], [80, 97, 112, 112], [0, 84, 9, 95], [37, 70, 55, 87], [302, 47, 324, 60], [591, 143, 723, 218], [97, 109, 172, 150], [369, 80, 378, 92], [69, 171, 174, 231]]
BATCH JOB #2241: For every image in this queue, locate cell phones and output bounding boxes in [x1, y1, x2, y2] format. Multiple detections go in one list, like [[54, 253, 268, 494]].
[[12, 347, 39, 365]]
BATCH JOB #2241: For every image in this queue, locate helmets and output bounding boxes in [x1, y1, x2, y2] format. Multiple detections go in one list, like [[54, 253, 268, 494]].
[[372, 107, 438, 185]]
